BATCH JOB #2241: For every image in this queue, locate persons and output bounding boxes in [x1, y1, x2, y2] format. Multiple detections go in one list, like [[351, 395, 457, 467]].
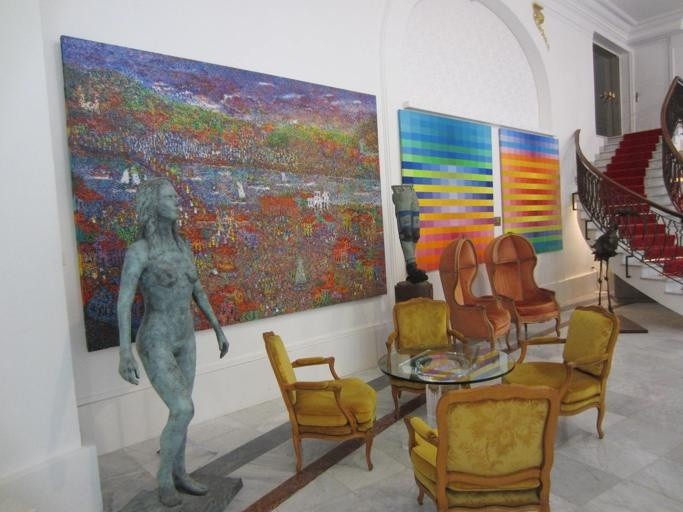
[[116, 178, 229, 507]]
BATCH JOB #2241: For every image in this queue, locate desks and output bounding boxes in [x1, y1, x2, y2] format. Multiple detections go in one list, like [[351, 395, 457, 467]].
[[377, 343, 518, 429]]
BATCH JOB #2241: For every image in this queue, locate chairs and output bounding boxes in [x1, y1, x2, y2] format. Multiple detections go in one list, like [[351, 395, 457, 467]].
[[385, 295, 470, 420], [261, 330, 379, 471], [438, 237, 513, 355], [500, 304, 623, 441], [402, 382, 563, 512], [484, 231, 561, 347]]
